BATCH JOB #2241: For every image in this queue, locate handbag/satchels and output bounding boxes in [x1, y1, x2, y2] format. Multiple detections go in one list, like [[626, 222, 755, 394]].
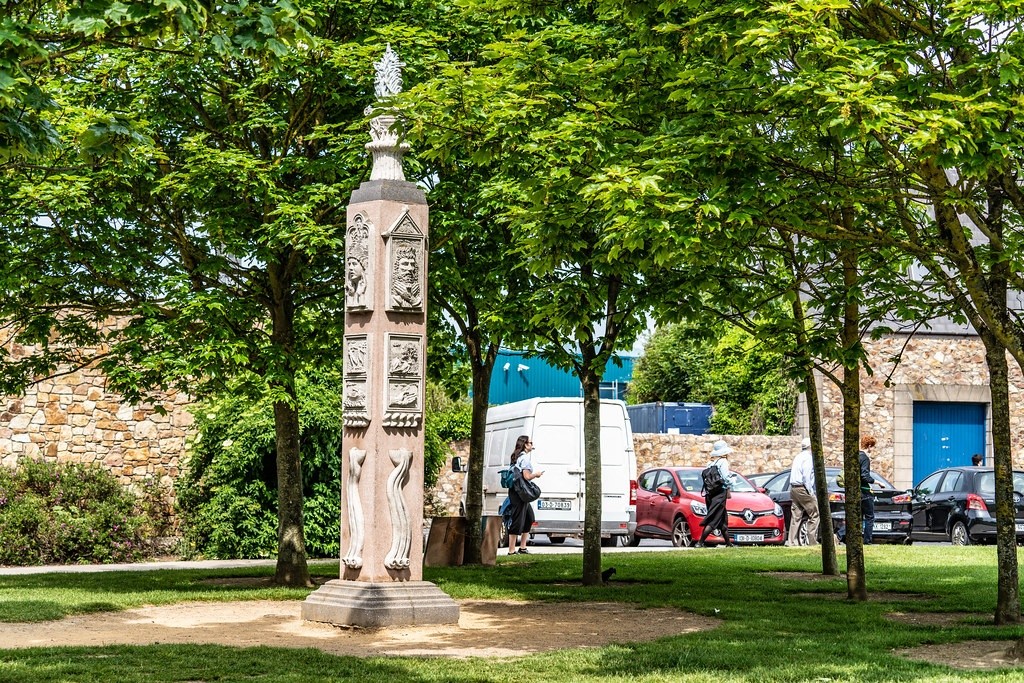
[[513, 466, 541, 504], [498, 469, 515, 488], [498, 497, 512, 524]]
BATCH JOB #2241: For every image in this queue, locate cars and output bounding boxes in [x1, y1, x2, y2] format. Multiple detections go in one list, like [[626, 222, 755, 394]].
[[620, 466, 787, 548], [758, 466, 914, 546], [732, 474, 792, 492]]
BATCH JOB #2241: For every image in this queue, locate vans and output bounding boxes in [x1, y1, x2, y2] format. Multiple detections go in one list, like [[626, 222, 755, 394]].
[[452, 396, 638, 548]]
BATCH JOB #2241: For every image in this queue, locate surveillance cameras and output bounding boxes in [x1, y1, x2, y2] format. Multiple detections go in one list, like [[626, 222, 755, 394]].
[[503, 363, 511, 371], [518, 364, 530, 370]]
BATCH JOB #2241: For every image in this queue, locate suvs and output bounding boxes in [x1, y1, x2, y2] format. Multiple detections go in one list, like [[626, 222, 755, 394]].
[[904, 466, 1024, 546]]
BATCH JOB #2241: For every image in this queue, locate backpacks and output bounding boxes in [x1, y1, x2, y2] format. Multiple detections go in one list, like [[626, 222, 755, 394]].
[[701, 457, 724, 497]]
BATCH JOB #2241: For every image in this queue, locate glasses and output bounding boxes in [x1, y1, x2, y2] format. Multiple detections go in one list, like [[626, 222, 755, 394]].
[[528, 442, 533, 445]]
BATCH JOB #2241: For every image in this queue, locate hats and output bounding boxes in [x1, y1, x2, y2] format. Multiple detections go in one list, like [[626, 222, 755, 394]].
[[801, 438, 811, 448], [711, 440, 733, 456]]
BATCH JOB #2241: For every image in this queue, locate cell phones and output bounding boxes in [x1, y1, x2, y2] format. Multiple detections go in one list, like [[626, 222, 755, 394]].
[[542, 470, 545, 473]]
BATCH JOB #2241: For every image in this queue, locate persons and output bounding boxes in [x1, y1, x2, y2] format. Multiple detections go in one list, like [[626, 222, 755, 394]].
[[347, 244, 368, 304], [507, 436, 543, 556], [392, 246, 423, 307], [695, 440, 739, 548], [785, 437, 820, 547], [834, 435, 885, 546], [972, 454, 984, 467]]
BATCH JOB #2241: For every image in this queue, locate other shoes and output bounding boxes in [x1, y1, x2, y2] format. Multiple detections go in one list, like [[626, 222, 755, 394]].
[[834, 534, 844, 545], [518, 548, 529, 554], [508, 551, 517, 555], [694, 542, 707, 548], [726, 544, 733, 547]]
